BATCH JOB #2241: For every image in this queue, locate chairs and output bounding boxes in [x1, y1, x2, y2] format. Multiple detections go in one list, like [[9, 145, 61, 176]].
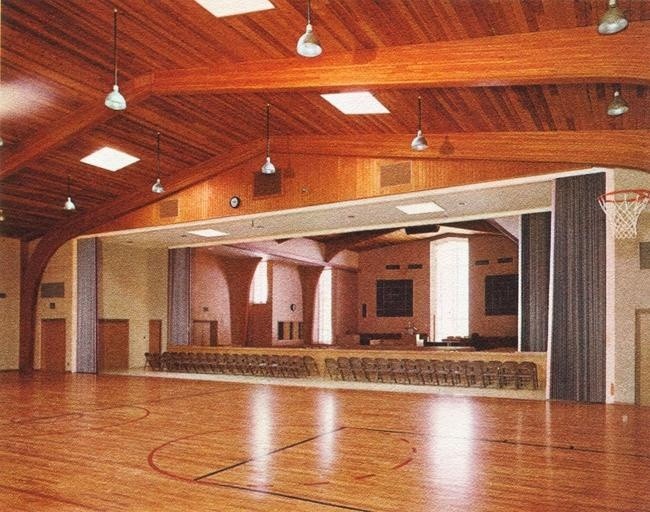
[[143, 350, 539, 394]]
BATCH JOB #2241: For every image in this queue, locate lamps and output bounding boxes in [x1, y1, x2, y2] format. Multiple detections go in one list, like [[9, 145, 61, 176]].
[[607, 84, 629, 117], [411, 96, 428, 151], [64, 175, 76, 210], [596, 0, 628, 34], [105, 10, 127, 111], [296, 0, 322, 57], [260, 104, 276, 175], [151, 132, 165, 194]]
